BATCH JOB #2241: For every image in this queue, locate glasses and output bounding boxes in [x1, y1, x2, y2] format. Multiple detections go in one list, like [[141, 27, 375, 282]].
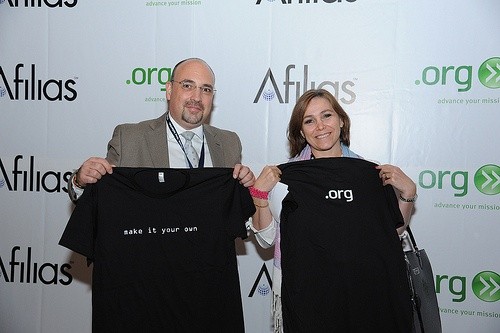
[[170, 79, 217, 96]]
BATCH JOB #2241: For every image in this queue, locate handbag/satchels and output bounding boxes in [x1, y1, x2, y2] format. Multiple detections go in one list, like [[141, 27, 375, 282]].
[[400, 225, 442, 333]]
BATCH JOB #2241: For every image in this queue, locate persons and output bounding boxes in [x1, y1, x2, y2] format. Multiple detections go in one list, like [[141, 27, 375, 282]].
[[68, 57, 257, 206], [244, 89, 418, 333]]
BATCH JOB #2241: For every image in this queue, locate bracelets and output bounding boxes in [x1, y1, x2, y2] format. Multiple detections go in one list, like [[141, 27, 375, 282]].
[[399, 194, 418, 202], [73, 170, 85, 189], [254, 202, 269, 207], [248, 186, 269, 200]]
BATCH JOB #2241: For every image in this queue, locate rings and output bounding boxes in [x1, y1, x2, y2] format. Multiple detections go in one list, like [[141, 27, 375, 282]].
[[384, 172, 388, 179]]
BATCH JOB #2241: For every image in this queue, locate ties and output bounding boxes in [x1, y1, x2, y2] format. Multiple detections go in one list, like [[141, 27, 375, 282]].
[[180, 131, 200, 168]]
